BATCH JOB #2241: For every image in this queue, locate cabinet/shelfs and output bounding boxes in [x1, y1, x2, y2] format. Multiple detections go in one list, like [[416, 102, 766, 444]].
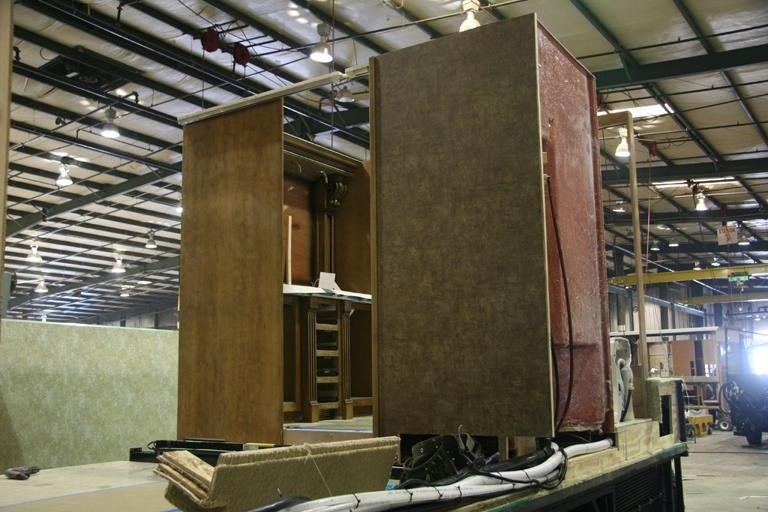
[[176, 13, 617, 465]]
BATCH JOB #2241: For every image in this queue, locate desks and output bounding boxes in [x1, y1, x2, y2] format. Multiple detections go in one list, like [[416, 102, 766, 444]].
[[1, 435, 688, 512]]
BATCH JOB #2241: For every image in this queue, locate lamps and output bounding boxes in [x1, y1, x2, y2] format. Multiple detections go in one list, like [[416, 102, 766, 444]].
[[55, 105, 120, 189], [111, 228, 158, 276], [613, 123, 632, 157], [460, 0, 482, 32], [696, 192, 709, 213], [25, 229, 49, 294], [309, 20, 334, 63]]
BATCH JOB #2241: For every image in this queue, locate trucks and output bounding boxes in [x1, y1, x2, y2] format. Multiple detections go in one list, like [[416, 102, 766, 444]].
[[725, 328, 768, 445]]
